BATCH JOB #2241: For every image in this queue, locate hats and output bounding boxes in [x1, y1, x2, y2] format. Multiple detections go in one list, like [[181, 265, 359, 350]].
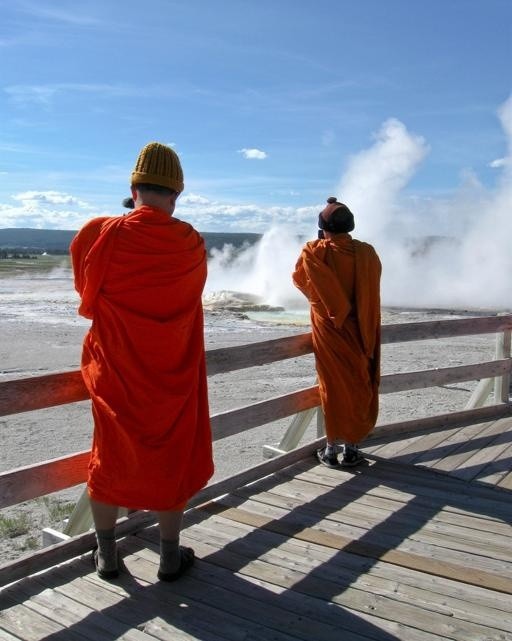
[[318, 197, 354, 233], [132, 143, 184, 193]]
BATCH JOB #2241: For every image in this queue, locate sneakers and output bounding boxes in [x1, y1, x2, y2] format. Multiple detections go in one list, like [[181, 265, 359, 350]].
[[316, 447, 339, 469], [340, 452, 361, 467]]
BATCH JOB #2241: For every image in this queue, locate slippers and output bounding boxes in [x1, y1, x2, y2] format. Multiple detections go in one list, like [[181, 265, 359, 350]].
[[158, 546, 195, 583], [94, 547, 119, 580]]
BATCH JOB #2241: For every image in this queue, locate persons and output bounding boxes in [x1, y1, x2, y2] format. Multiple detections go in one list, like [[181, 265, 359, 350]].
[[69, 142, 216, 583], [291, 197, 382, 469]]
